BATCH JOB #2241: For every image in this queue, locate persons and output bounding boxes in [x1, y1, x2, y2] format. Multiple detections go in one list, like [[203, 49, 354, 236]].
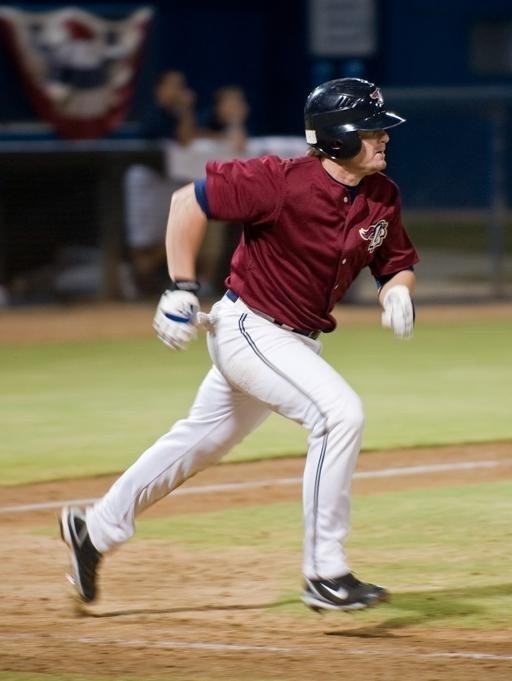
[[53, 78, 422, 615], [123, 66, 188, 300], [177, 78, 253, 298]]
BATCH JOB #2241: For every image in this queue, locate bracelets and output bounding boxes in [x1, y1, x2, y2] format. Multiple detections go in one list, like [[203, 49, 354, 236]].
[[171, 278, 202, 293]]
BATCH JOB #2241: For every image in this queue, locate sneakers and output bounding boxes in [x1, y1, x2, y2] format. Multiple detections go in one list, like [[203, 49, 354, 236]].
[[303, 573, 388, 611], [60, 505, 100, 601]]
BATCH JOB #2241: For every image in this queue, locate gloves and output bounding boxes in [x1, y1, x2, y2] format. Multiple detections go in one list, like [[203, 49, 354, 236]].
[[380, 284, 415, 335], [152, 279, 201, 351]]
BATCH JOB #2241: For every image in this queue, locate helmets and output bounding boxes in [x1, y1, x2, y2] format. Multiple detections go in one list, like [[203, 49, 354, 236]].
[[304, 77, 405, 159]]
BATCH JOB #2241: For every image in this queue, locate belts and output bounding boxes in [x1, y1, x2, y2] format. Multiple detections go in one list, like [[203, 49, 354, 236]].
[[226, 290, 320, 341]]
